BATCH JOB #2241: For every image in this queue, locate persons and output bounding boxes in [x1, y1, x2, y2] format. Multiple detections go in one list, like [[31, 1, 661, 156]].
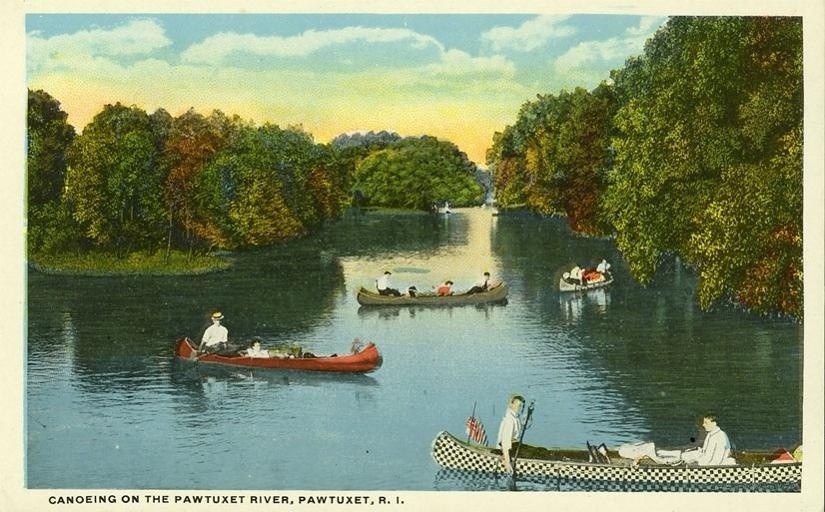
[[467, 272, 492, 293], [438, 280, 454, 296], [595, 256, 613, 281], [696, 412, 733, 465], [197, 312, 230, 353], [570, 264, 585, 285], [242, 339, 271, 358], [376, 271, 403, 297], [493, 394, 534, 477]]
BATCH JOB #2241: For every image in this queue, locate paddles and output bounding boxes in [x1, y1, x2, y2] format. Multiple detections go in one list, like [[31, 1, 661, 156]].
[[507, 400, 536, 490], [190, 349, 221, 363]]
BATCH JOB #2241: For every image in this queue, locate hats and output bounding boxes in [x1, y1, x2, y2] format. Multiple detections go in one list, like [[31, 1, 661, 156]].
[[210, 311, 225, 321]]
